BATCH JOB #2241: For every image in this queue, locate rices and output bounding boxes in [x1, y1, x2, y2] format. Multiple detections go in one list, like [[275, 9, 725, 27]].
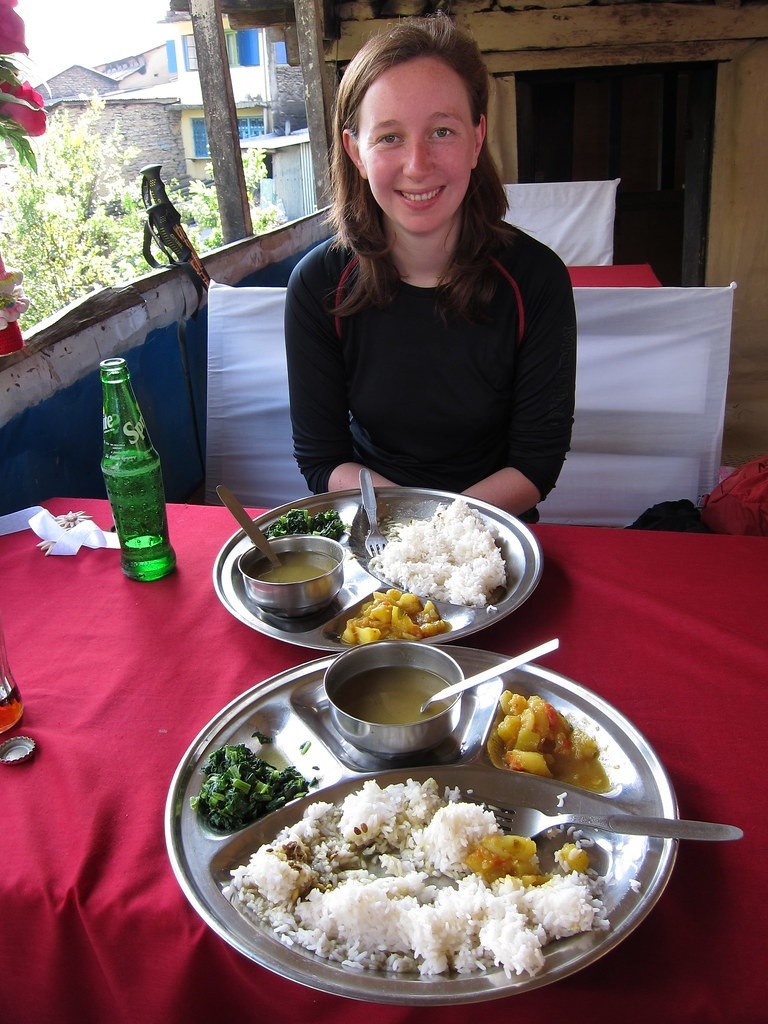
[[218, 778, 643, 977], [367, 498, 507, 614]]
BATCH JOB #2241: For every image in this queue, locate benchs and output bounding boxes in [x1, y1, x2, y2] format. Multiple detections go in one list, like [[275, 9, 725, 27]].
[[503, 176, 622, 266], [205, 280, 738, 528]]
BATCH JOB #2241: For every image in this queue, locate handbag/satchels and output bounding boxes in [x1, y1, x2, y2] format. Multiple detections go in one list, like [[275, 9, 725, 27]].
[[698, 456, 768, 536], [624, 499, 711, 534]]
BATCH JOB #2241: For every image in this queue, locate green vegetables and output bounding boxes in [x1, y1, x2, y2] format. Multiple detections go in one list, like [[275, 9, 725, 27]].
[[255, 509, 349, 540], [189, 732, 320, 832]]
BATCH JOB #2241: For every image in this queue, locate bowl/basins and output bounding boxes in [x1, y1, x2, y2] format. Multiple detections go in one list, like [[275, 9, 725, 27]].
[[237, 535, 344, 619], [324, 639, 465, 759]]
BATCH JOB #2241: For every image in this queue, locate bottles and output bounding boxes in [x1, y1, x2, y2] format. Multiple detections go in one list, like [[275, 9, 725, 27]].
[[0, 625, 24, 733], [100, 358, 177, 581]]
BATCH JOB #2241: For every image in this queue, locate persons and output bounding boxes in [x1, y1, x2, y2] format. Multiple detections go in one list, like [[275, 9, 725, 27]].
[[284, 19, 578, 524]]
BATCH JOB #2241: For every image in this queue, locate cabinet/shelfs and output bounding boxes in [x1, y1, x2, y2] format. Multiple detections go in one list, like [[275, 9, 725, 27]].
[[516, 71, 613, 180], [616, 72, 690, 211]]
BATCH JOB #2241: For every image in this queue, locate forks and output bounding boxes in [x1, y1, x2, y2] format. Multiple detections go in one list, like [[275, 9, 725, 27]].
[[456, 791, 744, 841], [359, 468, 387, 558]]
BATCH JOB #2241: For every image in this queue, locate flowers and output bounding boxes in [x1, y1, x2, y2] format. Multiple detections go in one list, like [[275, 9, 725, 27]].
[[0, 0, 48, 176]]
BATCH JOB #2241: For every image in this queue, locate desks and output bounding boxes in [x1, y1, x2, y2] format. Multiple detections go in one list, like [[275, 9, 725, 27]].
[[0, 500, 768, 1024], [570, 264, 662, 287]]
